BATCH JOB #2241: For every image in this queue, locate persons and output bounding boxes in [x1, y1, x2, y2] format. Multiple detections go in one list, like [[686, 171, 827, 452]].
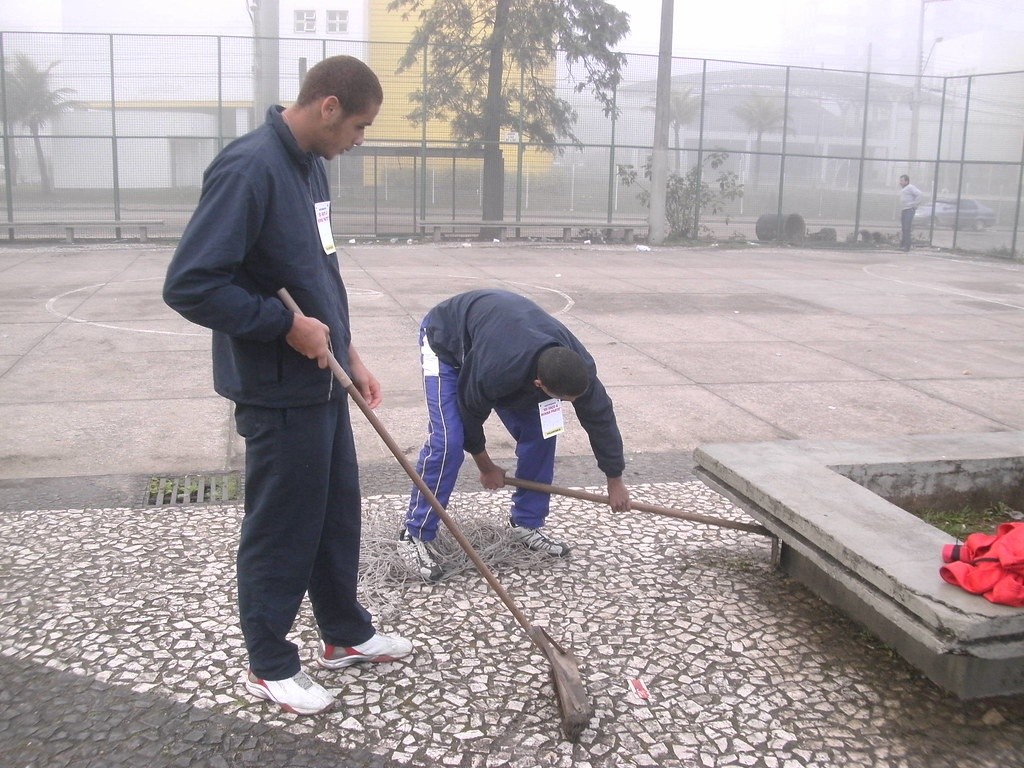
[[161, 54, 414, 718], [894, 174, 922, 253], [397, 287, 634, 581]]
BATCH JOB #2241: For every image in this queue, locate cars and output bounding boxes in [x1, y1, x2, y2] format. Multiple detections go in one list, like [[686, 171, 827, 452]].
[[913, 195, 998, 232]]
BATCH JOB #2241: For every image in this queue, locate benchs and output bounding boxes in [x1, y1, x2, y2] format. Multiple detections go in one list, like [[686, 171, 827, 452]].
[[417, 219, 648, 244], [0, 219, 164, 243]]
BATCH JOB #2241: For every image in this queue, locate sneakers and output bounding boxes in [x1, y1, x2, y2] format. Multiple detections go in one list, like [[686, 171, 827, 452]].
[[246, 653, 334, 715], [505, 517, 570, 556], [396, 529, 443, 580], [317, 627, 413, 669]]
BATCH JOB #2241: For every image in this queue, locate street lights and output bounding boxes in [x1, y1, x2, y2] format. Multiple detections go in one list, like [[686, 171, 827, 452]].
[[908, 0, 944, 186]]
